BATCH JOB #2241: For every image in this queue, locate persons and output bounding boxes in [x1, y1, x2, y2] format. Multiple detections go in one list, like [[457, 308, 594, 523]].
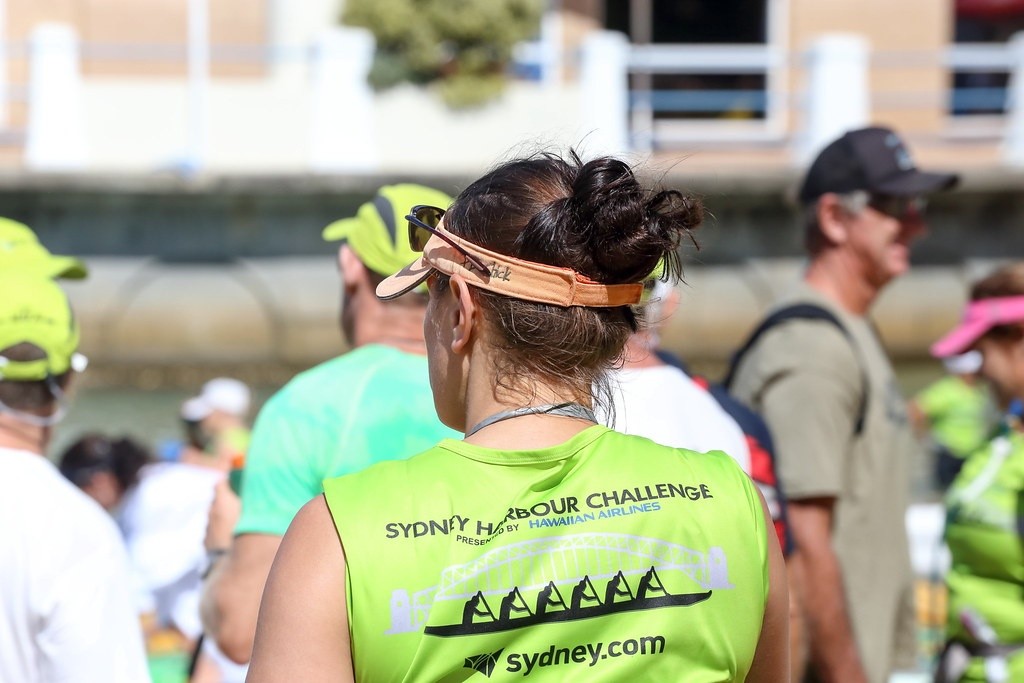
[[0, 127, 1023, 682], [246, 150, 790, 682]]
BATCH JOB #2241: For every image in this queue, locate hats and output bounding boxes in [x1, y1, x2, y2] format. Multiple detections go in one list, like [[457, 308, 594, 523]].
[[800, 127, 958, 204], [927, 294, 1024, 358], [375, 212, 645, 309], [322, 183, 453, 294], [181, 377, 250, 423], [0, 216, 89, 381]]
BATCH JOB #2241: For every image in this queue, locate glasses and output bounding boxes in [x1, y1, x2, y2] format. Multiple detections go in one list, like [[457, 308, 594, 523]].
[[867, 191, 937, 223], [405, 205, 491, 278]]
